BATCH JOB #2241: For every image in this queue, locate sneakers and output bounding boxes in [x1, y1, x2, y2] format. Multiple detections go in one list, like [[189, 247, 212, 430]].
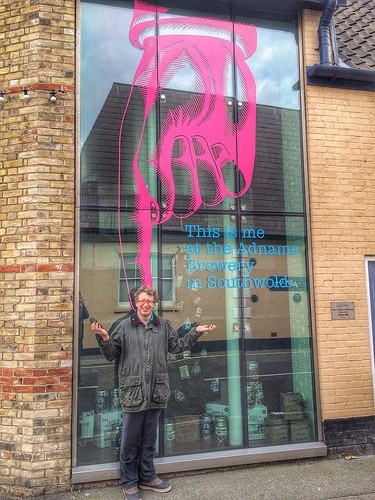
[[138, 478, 171, 492], [123, 484, 139, 500]]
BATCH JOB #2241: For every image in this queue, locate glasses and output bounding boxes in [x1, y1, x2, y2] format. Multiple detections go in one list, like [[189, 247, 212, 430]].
[[137, 300, 155, 304]]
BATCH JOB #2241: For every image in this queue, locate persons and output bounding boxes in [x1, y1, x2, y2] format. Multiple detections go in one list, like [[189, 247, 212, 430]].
[[91, 285, 216, 500], [79, 293, 89, 385], [89, 286, 203, 460]]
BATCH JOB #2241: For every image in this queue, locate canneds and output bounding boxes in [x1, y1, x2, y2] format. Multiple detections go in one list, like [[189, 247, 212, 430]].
[[253, 382, 265, 407], [163, 418, 176, 448], [200, 413, 214, 439], [108, 388, 121, 412], [246, 383, 255, 409], [95, 389, 109, 412], [246, 361, 259, 386], [214, 415, 229, 442]]
